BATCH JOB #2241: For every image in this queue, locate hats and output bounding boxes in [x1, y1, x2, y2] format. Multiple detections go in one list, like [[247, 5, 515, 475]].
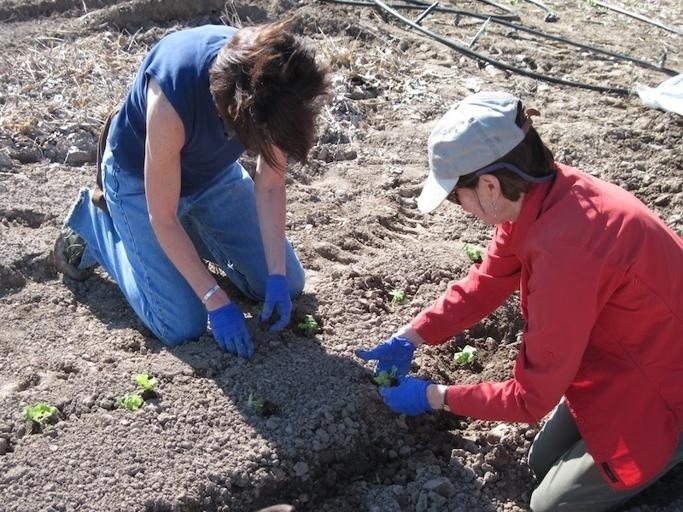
[[417, 90, 540, 214]]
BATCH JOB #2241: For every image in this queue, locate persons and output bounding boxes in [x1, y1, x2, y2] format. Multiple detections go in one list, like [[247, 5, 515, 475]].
[[350, 87, 683, 510], [49, 20, 333, 359]]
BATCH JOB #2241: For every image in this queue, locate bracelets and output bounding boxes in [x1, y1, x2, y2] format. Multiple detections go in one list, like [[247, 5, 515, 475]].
[[200, 285, 221, 307]]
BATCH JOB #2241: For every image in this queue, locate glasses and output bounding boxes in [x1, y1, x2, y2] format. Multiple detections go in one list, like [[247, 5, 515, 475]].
[[447, 173, 485, 205]]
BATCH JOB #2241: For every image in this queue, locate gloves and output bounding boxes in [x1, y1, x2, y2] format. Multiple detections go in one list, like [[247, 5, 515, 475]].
[[378, 375, 435, 417], [262, 274, 293, 331], [355, 335, 416, 376], [205, 301, 254, 358]]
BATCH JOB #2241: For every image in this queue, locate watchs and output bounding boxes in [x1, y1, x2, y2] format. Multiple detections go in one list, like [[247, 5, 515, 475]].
[[441, 386, 451, 413]]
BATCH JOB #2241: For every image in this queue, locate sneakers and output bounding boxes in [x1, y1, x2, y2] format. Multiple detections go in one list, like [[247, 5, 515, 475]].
[[53, 199, 97, 280]]
